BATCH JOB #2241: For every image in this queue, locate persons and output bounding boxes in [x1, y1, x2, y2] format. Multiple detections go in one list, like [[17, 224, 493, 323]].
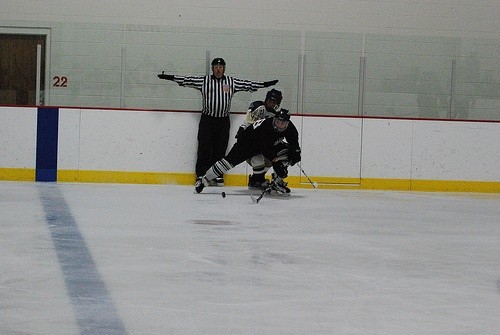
[[195, 109, 301, 193], [235, 88, 283, 190], [157, 58, 279, 186]]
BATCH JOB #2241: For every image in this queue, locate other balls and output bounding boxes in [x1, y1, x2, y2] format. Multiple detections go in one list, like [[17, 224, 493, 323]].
[[221, 191, 226, 198]]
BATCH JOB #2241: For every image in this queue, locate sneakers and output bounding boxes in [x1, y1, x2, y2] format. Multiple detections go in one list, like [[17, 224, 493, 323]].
[[195, 177, 209, 193], [248, 173, 269, 190], [216, 178, 224, 186], [270, 173, 291, 196]]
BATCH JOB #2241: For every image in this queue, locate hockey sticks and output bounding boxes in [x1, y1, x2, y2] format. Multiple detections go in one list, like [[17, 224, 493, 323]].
[[296, 162, 319, 189], [249, 160, 293, 204]]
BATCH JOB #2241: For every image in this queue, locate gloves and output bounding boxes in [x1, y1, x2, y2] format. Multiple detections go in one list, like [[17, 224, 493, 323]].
[[288, 147, 301, 166], [264, 80, 279, 87], [157, 71, 174, 81]]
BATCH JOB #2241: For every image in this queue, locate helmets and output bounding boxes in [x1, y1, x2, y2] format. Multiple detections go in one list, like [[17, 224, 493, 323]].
[[211, 58, 225, 66], [275, 108, 290, 122], [266, 89, 283, 105]]
